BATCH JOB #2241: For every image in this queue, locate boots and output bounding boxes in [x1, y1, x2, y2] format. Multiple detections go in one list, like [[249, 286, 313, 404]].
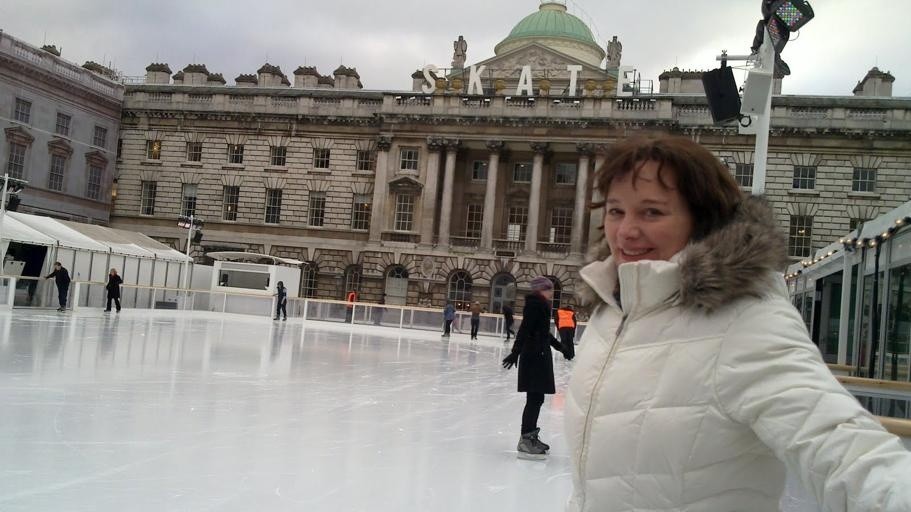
[[283, 316, 287, 321], [517, 427, 550, 455], [273, 315, 280, 320]]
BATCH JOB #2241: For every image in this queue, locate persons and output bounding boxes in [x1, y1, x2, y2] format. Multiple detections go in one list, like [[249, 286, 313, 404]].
[[471, 300, 485, 339], [373, 293, 388, 326], [103, 268, 123, 311], [555, 306, 576, 353], [501, 275, 574, 455], [345, 290, 356, 323], [503, 305, 517, 343], [568, 133, 911, 512], [45, 262, 71, 311], [442, 299, 456, 336], [273, 282, 288, 321]]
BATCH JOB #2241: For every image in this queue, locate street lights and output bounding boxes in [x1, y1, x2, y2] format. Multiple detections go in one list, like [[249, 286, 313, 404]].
[[701, 0, 814, 199], [0, 173, 28, 276], [176, 214, 205, 289]]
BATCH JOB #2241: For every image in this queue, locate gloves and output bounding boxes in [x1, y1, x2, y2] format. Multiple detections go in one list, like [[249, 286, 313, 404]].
[[502, 352, 518, 370], [561, 345, 572, 360]]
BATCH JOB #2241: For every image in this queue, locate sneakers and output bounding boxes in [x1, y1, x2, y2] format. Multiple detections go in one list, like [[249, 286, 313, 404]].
[[104, 309, 111, 312], [57, 305, 66, 312], [116, 307, 121, 312], [441, 332, 516, 342]]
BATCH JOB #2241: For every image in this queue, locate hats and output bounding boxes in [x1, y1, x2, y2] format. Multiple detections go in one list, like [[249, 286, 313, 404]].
[[531, 276, 553, 291], [107, 268, 118, 272], [475, 301, 480, 305], [447, 299, 451, 304]]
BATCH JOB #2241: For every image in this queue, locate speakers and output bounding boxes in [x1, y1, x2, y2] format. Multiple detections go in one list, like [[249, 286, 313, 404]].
[[739, 69, 773, 115], [701, 64, 741, 124], [194, 230, 203, 243], [5, 196, 21, 212]]
